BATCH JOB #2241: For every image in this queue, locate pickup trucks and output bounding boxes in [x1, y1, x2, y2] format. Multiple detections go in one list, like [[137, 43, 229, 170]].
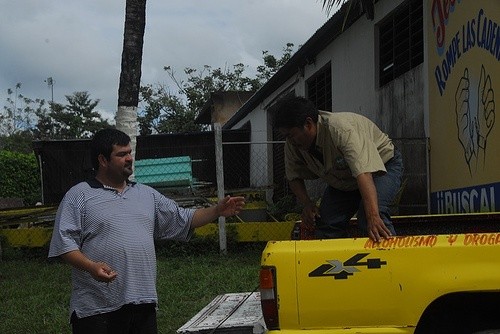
[[176, 212, 500, 334]]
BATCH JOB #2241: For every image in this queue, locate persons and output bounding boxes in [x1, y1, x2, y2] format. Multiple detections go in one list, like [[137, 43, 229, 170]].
[[48, 129, 246, 334], [274, 96, 405, 243]]
